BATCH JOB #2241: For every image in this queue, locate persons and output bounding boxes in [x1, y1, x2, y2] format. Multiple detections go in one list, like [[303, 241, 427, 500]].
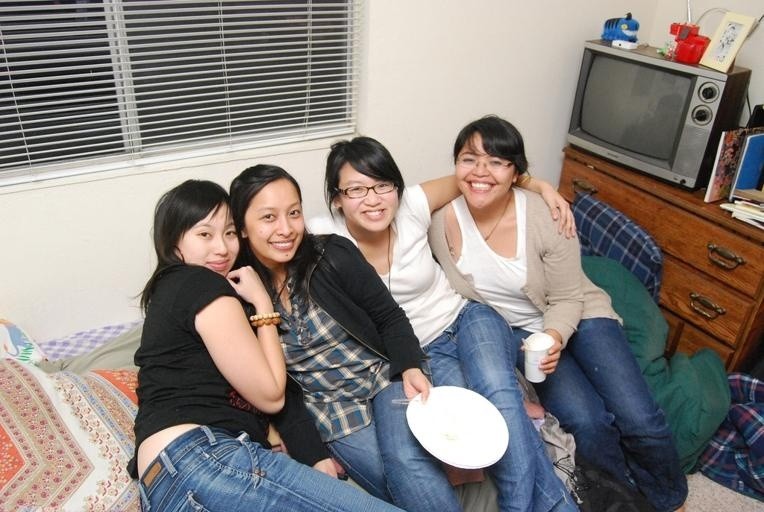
[[229, 163, 467, 512], [425, 114, 691, 512], [300, 135, 586, 512], [131, 178, 405, 511]]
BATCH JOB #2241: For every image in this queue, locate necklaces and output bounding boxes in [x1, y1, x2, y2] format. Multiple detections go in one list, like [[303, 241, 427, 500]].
[[483, 188, 513, 241], [272, 278, 290, 305], [385, 223, 393, 292]]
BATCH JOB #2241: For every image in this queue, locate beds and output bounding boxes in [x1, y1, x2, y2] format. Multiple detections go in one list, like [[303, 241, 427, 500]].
[[1, 316, 600, 512]]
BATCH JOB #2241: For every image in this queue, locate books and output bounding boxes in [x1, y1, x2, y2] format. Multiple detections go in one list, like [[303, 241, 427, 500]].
[[702, 125, 763, 230]]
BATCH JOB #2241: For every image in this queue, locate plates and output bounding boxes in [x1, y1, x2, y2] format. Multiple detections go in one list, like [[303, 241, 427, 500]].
[[407, 386, 509, 471]]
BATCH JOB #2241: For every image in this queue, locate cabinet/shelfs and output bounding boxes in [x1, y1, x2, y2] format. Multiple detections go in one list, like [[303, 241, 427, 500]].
[[558, 144, 764, 376]]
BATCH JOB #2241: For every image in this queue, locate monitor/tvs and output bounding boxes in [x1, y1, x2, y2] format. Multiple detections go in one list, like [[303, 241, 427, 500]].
[[567, 41, 751, 191]]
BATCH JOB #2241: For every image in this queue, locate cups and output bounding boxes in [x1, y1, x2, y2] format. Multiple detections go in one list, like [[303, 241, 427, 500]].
[[524, 333, 555, 384]]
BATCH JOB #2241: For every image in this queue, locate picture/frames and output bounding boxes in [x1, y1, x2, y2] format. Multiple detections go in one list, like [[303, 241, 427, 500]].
[[698, 12, 756, 73]]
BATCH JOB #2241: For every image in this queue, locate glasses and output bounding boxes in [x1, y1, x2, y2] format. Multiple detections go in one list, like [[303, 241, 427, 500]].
[[455, 152, 514, 170], [334, 182, 395, 200]]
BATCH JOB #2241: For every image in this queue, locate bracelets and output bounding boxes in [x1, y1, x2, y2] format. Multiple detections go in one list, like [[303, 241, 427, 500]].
[[250, 316, 282, 327], [249, 311, 280, 321]]
